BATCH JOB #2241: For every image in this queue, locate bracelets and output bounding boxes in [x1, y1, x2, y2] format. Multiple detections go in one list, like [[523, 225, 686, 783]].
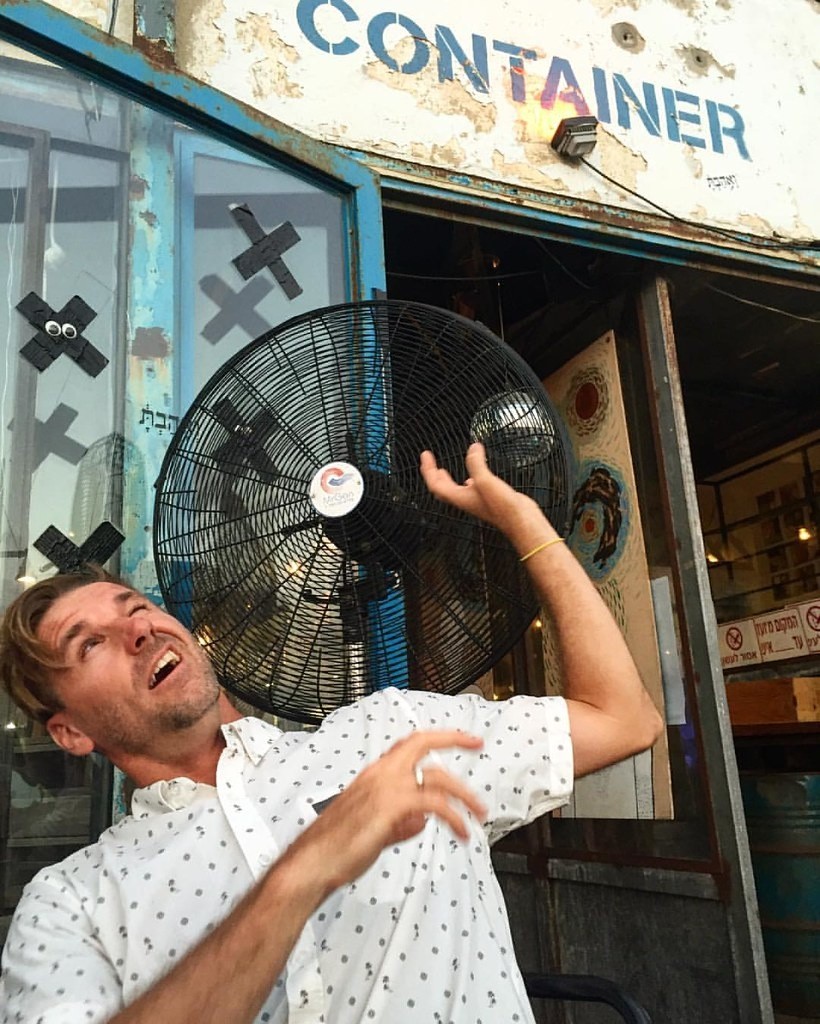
[[520, 537, 566, 563]]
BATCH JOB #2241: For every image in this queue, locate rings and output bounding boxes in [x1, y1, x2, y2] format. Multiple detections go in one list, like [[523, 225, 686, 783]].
[[414, 767, 425, 792]]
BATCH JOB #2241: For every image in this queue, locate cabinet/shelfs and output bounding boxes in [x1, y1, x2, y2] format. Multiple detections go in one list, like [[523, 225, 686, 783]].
[[693, 438, 820, 624]]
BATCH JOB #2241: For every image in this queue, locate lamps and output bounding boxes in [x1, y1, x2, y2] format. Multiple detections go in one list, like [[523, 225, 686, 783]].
[[796, 528, 814, 543], [706, 553, 719, 564]]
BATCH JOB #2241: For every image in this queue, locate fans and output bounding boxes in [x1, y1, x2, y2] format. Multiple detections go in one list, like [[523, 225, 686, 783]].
[[154, 293, 573, 726]]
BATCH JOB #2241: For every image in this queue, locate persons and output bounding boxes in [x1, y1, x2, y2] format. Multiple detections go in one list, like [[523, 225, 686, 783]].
[[1, 438, 667, 1024]]
